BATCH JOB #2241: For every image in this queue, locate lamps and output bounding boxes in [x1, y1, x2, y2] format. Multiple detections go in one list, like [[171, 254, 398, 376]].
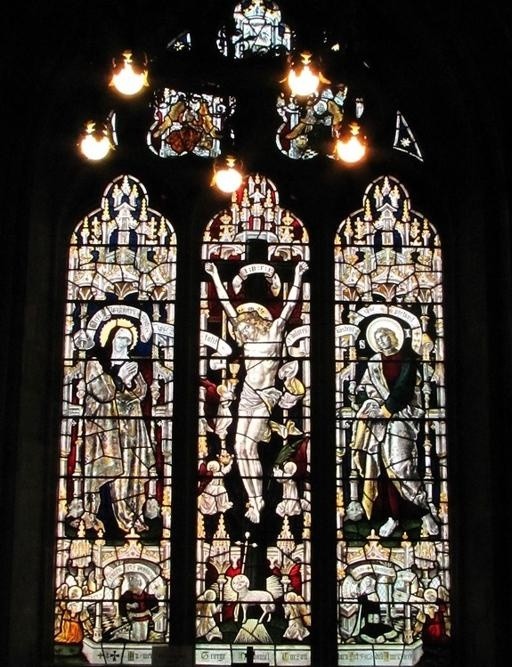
[[79, 107, 116, 163], [329, 124, 365, 166], [280, 48, 328, 99], [209, 154, 248, 194], [107, 48, 149, 98]]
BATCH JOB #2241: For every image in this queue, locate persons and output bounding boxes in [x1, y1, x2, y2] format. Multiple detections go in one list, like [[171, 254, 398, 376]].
[[201, 257, 311, 525], [117, 575, 160, 643], [72, 326, 155, 534], [348, 324, 439, 521]]
